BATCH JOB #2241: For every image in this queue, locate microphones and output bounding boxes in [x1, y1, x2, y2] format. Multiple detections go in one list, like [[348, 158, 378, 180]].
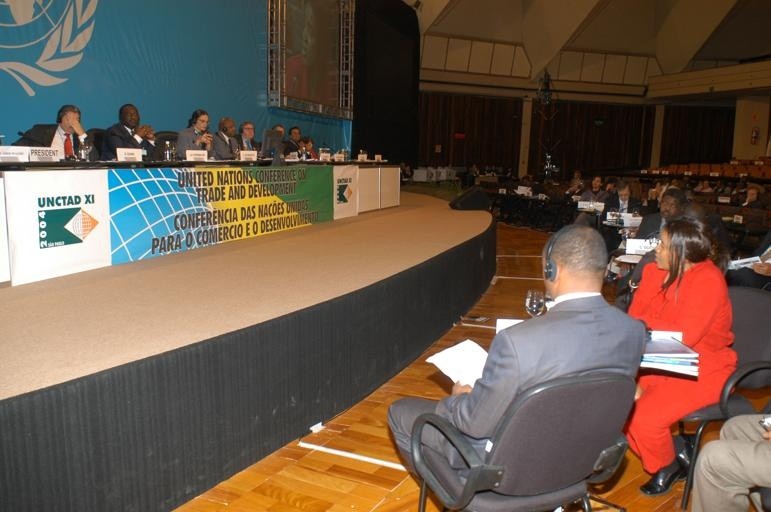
[[17, 131, 47, 147]]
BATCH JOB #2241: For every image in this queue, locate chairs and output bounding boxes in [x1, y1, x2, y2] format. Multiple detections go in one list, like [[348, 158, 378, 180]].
[[410, 364, 637, 511], [155, 130, 179, 160], [85, 127, 104, 160], [679, 286, 771, 512], [604, 174, 771, 261], [646, 155, 771, 177]]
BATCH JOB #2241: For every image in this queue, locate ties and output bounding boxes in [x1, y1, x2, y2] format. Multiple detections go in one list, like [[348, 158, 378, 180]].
[[245, 141, 252, 150], [229, 139, 232, 153], [64, 132, 74, 158], [131, 131, 135, 135]]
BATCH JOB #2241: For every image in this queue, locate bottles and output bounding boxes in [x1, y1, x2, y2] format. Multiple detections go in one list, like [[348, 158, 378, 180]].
[[301, 146, 308, 161], [78, 138, 88, 163], [164, 140, 173, 162]]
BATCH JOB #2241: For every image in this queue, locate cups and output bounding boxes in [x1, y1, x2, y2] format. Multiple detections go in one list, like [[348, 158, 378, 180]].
[[524, 288, 543, 318]]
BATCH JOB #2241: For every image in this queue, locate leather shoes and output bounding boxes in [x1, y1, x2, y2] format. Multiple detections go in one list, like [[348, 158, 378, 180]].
[[640, 457, 688, 496], [676, 433, 699, 481]]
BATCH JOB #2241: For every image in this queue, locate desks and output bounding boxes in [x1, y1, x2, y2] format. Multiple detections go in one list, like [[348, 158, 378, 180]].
[[484, 191, 579, 233]]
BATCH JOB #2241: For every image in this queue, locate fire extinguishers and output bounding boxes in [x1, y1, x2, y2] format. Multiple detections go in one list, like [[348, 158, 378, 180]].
[[750, 128, 760, 145]]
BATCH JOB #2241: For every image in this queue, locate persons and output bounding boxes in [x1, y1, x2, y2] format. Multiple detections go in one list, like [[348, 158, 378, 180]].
[[10, 105, 100, 161], [238, 121, 255, 151], [95, 103, 162, 161], [272, 124, 318, 159], [212, 117, 239, 160], [387, 161, 771, 512], [176, 109, 213, 159]]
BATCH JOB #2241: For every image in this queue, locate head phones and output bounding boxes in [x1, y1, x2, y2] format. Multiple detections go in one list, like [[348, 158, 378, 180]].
[[544, 226, 574, 281], [223, 127, 227, 133]]
